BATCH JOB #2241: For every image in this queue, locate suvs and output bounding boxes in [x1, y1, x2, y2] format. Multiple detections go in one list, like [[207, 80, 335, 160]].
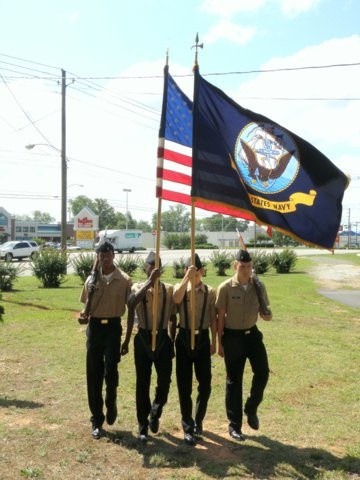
[[0, 241, 40, 263]]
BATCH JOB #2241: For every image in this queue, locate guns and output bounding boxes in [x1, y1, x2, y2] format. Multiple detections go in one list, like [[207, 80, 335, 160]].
[[84, 225, 107, 318], [236, 227, 270, 315]]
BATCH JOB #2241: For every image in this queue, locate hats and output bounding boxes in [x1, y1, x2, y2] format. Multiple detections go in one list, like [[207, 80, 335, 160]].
[[145, 251, 161, 265], [98, 241, 113, 253], [185, 253, 202, 270], [236, 249, 252, 262]]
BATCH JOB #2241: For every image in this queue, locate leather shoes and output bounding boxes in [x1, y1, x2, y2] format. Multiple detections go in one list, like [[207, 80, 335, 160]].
[[105, 408, 117, 425], [91, 426, 102, 439], [195, 422, 202, 434], [184, 432, 196, 445], [244, 408, 259, 430], [138, 434, 147, 442], [149, 415, 159, 434], [230, 430, 245, 441]]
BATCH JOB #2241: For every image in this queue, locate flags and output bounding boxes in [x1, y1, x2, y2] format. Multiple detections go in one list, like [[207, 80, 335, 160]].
[[192, 65, 350, 252], [156, 65, 272, 236]]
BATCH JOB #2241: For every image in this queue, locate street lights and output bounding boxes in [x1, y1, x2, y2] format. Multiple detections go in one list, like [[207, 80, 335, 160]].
[[124, 188, 132, 229], [25, 143, 67, 275]]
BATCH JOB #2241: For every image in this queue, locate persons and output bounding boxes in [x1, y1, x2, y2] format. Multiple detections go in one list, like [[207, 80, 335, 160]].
[[128, 251, 177, 441], [79, 242, 132, 439], [216, 250, 272, 439], [172, 254, 217, 447]]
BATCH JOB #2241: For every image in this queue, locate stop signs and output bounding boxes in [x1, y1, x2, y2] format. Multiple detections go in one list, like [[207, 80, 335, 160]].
[[152, 230, 156, 236]]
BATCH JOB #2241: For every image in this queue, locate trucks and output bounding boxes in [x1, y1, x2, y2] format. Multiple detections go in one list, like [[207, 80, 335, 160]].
[[95, 229, 147, 254]]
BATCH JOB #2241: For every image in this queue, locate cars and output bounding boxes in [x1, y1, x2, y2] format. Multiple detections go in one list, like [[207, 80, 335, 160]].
[[43, 240, 81, 251]]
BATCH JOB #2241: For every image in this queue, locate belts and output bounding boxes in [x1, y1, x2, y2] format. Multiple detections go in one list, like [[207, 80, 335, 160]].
[[179, 328, 208, 335], [89, 316, 121, 324], [224, 327, 257, 335], [138, 327, 167, 335]]
